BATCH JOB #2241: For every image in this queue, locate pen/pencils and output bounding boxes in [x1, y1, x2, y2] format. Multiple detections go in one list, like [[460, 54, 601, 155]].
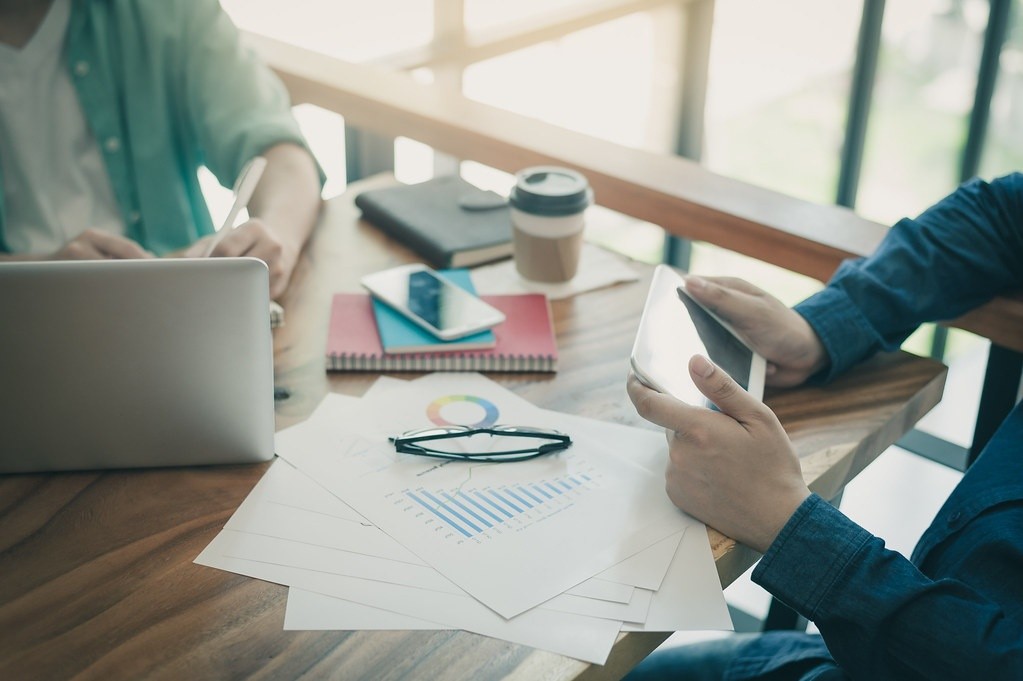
[[199, 152, 272, 259]]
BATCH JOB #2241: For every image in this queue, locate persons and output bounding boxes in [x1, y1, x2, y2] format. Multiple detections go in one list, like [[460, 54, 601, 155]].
[[624, 168, 1023, 681], [0, 0, 327, 300]]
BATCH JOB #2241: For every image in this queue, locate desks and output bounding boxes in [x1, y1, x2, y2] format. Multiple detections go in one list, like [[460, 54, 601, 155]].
[[1, 173, 950, 680]]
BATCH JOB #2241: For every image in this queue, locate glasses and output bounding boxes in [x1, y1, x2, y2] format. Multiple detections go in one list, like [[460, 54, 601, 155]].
[[388, 424, 573, 463]]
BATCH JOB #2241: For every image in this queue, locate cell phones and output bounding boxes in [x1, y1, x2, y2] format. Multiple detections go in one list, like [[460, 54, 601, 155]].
[[359, 263, 506, 340]]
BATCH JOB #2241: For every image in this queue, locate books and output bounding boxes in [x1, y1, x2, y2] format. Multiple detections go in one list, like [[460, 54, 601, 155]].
[[371, 268, 497, 355], [322, 293, 558, 372], [355, 173, 515, 269]]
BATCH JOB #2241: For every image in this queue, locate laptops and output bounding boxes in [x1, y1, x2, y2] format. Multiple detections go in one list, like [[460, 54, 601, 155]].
[[0, 261, 274, 473]]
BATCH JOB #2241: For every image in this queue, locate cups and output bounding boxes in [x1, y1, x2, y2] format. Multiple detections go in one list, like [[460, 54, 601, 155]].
[[509, 166, 594, 282]]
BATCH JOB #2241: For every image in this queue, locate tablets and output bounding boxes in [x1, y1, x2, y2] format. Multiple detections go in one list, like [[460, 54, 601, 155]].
[[630, 264, 767, 415]]
